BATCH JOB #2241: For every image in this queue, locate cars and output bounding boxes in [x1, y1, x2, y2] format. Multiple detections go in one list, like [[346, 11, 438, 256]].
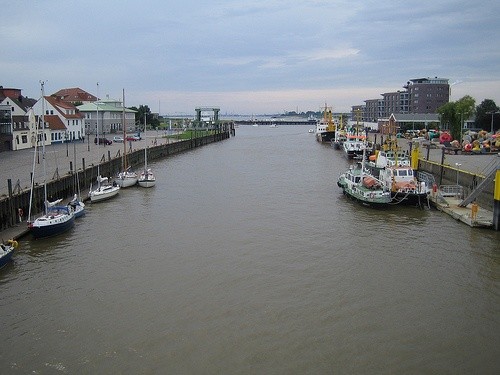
[[126, 135, 137, 142], [113, 136, 124, 142], [134, 135, 142, 140]]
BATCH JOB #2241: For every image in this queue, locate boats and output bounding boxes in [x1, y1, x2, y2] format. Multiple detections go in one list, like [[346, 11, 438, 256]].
[[0, 237, 19, 267], [308, 101, 430, 210], [270, 124, 278, 128]]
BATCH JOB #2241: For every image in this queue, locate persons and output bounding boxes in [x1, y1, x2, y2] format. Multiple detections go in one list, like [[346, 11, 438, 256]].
[[18, 208, 24, 222], [452, 140, 458, 155]]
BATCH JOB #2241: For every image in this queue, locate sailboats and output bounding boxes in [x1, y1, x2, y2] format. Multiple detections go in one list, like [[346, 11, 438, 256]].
[[63, 163, 86, 218], [86, 82, 121, 203], [115, 88, 140, 188], [17, 79, 76, 237], [136, 112, 157, 188]]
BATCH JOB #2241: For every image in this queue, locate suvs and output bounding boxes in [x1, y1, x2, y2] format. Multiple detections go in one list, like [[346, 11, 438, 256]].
[[93, 137, 113, 146]]
